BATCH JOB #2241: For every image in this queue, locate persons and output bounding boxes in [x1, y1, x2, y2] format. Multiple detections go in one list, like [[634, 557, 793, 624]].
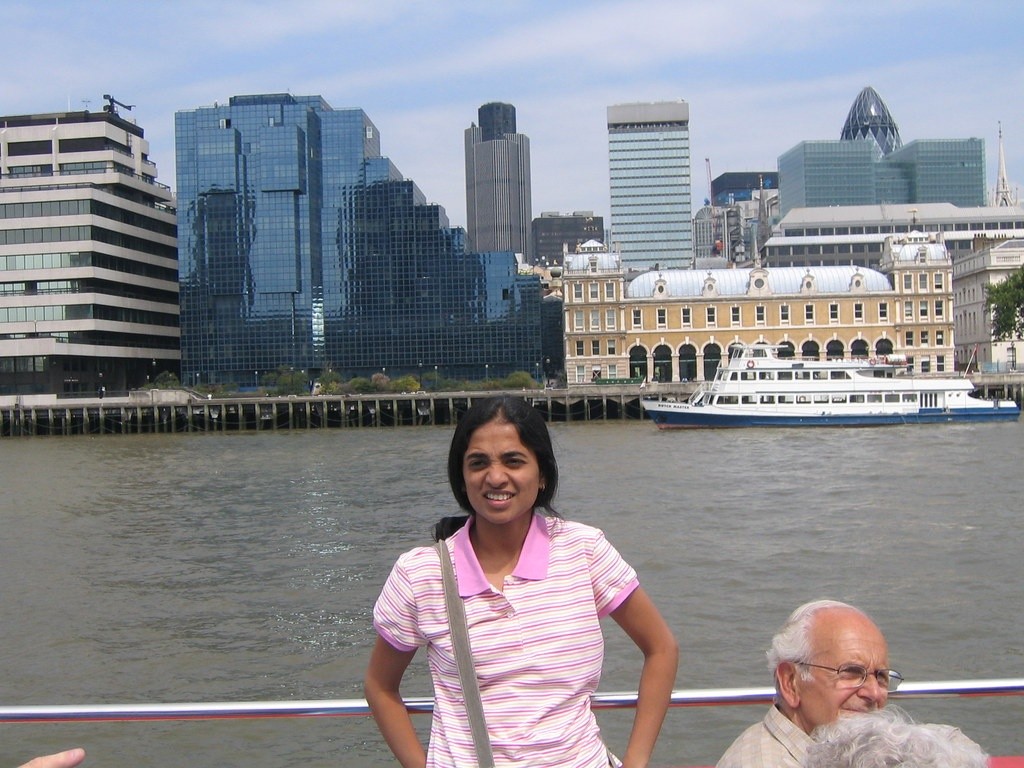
[[716, 598, 990, 767], [364, 391, 680, 767]]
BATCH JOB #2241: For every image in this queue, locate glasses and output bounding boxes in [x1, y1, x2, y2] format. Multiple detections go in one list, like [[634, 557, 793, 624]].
[[795, 660, 904, 692]]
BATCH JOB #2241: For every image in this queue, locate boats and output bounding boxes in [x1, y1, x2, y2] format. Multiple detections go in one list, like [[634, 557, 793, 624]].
[[640, 343, 1021, 429]]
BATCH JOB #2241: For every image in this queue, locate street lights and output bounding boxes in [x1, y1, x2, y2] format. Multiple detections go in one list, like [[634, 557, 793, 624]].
[[418, 360, 423, 391], [536, 361, 540, 381], [485, 363, 489, 383], [383, 367, 386, 380], [433, 364, 439, 386], [254, 370, 258, 392], [545, 356, 551, 384], [97, 372, 106, 399], [152, 359, 158, 389]]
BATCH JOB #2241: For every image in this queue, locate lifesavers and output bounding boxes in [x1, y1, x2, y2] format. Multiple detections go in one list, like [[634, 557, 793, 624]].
[[869, 359, 877, 365], [798, 395, 806, 402], [747, 360, 755, 368]]
